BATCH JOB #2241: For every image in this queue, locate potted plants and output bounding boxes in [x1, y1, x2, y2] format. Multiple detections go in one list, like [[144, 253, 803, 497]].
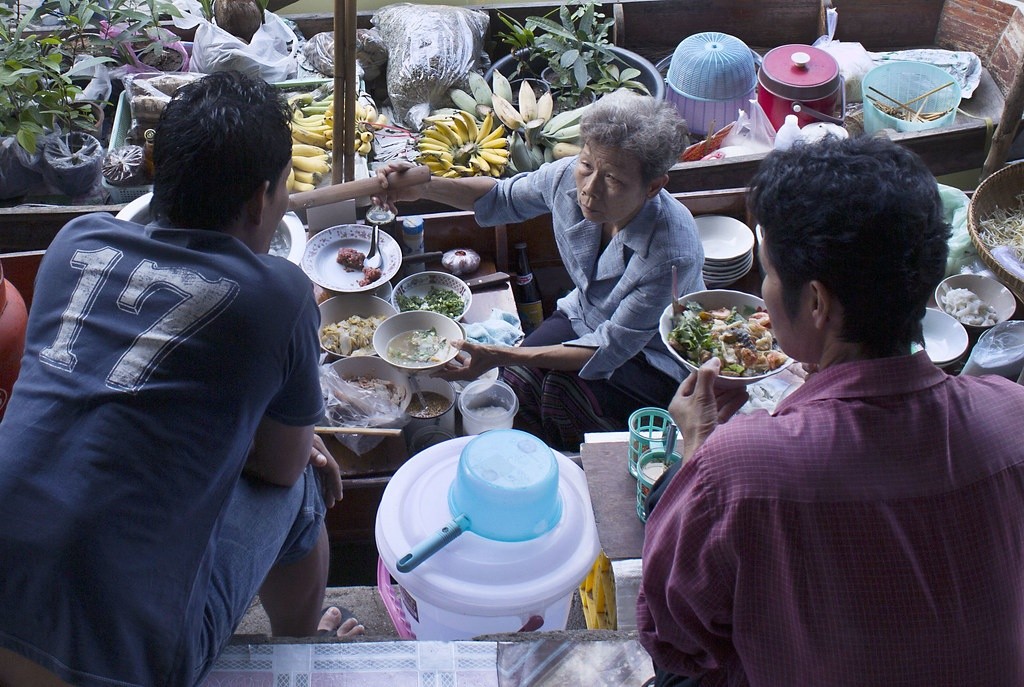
[[482, 2, 667, 141], [0, 0, 188, 192]]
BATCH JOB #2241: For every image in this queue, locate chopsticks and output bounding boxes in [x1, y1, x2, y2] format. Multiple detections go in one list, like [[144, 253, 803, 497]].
[[866, 81, 954, 123]]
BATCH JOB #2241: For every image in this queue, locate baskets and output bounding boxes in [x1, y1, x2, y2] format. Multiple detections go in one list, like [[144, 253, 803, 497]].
[[967, 162, 1024, 304], [862, 62, 962, 135], [667, 32, 758, 102], [664, 83, 756, 135]]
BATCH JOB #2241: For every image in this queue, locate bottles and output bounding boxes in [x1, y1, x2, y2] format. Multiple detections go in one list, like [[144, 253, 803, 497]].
[[513, 242, 544, 335], [364, 204, 398, 245], [773, 114, 802, 151], [142, 128, 157, 181], [398, 216, 426, 281]]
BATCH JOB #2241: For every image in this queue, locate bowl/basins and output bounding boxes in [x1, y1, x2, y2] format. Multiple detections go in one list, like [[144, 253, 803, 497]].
[[317, 293, 399, 361], [658, 289, 795, 390], [325, 356, 412, 427], [391, 271, 473, 322], [910, 307, 969, 370], [935, 273, 1017, 329], [373, 311, 467, 376], [693, 214, 755, 288]]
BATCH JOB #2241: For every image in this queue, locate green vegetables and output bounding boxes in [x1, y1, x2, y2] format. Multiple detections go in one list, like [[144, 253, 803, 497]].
[[667, 298, 770, 377]]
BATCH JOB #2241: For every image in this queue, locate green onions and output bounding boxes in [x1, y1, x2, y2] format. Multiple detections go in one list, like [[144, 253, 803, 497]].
[[394, 286, 464, 320]]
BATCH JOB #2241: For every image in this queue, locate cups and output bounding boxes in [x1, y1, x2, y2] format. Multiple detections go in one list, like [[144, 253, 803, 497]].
[[406, 375, 456, 457], [458, 378, 519, 442]]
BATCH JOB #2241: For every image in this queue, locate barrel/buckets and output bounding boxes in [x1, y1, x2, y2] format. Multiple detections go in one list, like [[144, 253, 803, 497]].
[[860, 61, 961, 138], [374, 434, 602, 641], [757, 44, 847, 138]]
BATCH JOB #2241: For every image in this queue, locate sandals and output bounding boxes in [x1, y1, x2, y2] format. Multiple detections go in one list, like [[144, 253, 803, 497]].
[[316, 606, 364, 638]]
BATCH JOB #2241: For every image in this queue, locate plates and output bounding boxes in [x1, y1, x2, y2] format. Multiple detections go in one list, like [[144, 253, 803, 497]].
[[362, 281, 393, 303], [446, 346, 499, 395], [301, 223, 402, 293], [801, 122, 849, 144]]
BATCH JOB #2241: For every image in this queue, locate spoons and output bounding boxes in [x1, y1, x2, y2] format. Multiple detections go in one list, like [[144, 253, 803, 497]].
[[671, 265, 690, 317], [362, 224, 383, 269]]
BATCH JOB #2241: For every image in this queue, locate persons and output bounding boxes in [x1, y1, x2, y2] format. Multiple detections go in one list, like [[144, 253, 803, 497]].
[[0, 69, 364, 686], [372, 96, 706, 453], [636, 132, 1024, 687]]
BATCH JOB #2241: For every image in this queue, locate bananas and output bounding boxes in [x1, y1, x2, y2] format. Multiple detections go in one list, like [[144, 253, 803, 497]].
[[285, 92, 386, 194], [414, 68, 590, 178]]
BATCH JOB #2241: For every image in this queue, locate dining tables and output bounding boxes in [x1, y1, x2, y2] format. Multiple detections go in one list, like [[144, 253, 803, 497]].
[[289, 274, 528, 478]]
